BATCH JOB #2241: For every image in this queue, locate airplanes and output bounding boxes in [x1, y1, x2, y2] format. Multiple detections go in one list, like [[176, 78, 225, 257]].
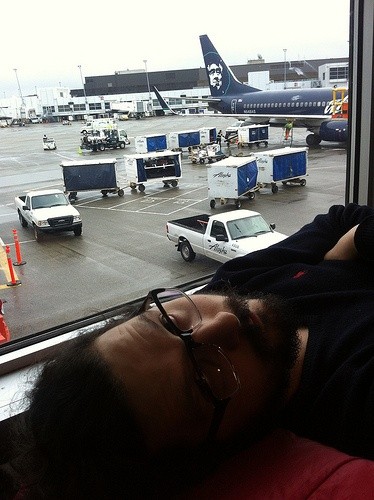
[[151, 34, 349, 147]]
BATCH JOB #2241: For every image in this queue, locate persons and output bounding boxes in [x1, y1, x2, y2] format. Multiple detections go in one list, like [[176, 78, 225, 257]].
[[43, 134, 47, 143], [282, 121, 292, 140], [7, 202, 374, 500], [216, 130, 225, 147]]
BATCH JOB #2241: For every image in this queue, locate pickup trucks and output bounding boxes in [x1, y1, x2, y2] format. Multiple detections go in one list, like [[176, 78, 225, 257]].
[[15, 188, 83, 240], [166, 208, 289, 264]]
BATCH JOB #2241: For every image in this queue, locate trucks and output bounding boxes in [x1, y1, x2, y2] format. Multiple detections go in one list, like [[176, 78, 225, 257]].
[[78, 122, 131, 152]]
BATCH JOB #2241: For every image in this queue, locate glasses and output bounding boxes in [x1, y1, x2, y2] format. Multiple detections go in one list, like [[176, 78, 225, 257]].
[[129, 288, 243, 452]]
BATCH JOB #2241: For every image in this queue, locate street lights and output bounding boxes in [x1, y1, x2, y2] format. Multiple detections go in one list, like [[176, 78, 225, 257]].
[[12, 67, 26, 106], [76, 64, 88, 104], [142, 58, 151, 100], [283, 48, 287, 89]]
